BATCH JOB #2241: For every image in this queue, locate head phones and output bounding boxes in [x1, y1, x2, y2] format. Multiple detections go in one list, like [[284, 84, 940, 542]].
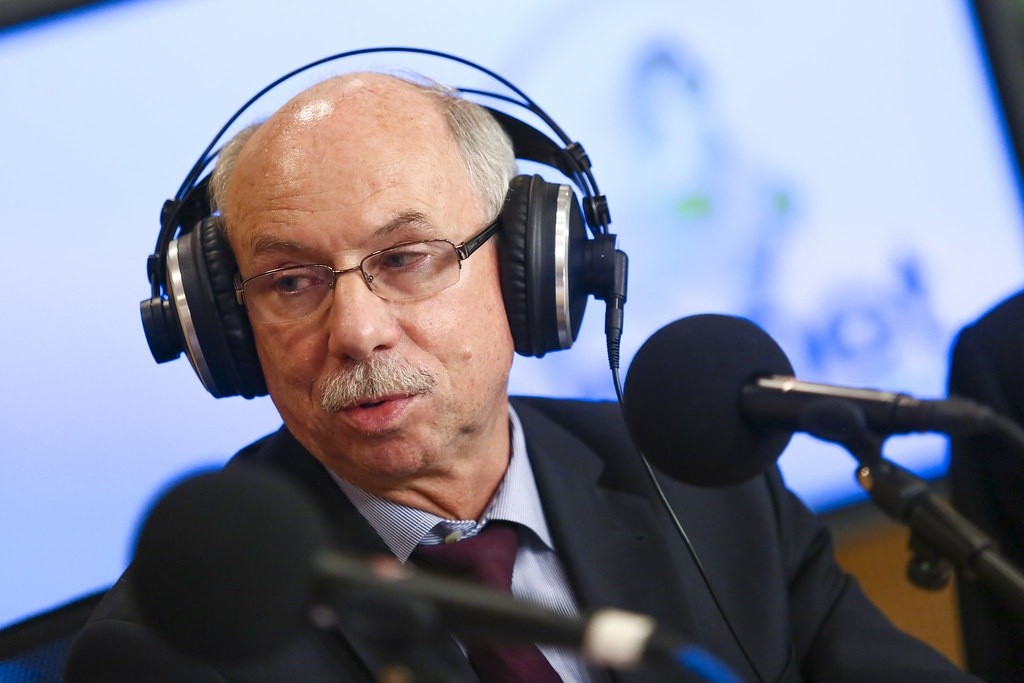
[[139, 46, 625, 401]]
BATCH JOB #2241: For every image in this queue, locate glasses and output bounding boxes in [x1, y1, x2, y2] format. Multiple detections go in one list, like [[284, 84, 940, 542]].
[[230, 216, 501, 324]]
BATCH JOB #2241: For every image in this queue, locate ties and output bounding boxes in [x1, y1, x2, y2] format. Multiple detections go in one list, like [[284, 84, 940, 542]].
[[414, 523, 567, 681]]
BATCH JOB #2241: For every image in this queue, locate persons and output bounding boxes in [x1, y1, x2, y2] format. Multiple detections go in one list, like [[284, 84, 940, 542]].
[[64, 74, 991, 681], [947, 288, 1022, 682]]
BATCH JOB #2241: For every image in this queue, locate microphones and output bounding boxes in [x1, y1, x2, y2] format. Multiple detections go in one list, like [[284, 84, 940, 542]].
[[622, 313, 992, 488], [131, 463, 680, 667]]
